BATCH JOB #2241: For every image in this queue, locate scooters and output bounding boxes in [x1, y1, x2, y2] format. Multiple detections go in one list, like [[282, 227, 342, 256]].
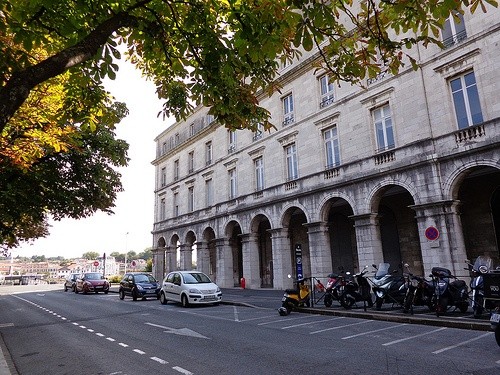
[[281, 274, 313, 314], [323, 255, 500, 344]]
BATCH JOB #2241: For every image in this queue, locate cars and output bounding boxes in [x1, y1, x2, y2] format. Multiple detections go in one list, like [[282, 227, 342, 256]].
[[159, 270, 223, 308], [64, 273, 80, 292], [75, 272, 110, 294], [119, 271, 161, 302]]
